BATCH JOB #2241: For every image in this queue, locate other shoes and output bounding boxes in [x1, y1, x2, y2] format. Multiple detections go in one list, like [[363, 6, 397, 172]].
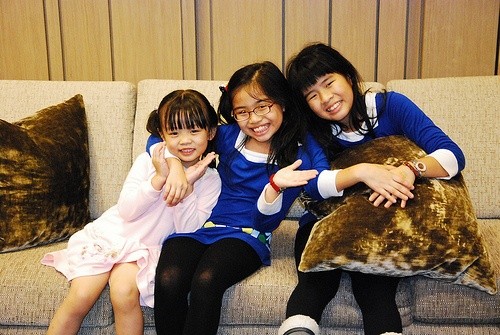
[[277, 314, 320, 335]]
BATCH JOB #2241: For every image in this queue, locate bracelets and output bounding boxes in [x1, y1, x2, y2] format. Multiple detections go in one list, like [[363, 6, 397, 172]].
[[269, 174, 286, 194], [406, 161, 426, 179]]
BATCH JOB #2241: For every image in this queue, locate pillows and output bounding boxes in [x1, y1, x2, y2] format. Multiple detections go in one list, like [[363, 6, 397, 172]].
[[297, 137, 497, 295], [0, 94, 91, 255]]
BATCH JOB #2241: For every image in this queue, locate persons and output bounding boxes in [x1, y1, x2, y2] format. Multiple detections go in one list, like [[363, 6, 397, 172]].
[[41, 89, 223, 335], [277, 43, 466, 335], [145, 61, 330, 335]]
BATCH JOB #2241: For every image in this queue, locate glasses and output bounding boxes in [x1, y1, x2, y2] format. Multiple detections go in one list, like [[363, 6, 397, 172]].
[[231, 100, 276, 121]]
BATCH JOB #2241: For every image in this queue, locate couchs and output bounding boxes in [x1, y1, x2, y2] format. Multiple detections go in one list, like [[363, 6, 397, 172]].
[[0, 75, 500, 335]]
